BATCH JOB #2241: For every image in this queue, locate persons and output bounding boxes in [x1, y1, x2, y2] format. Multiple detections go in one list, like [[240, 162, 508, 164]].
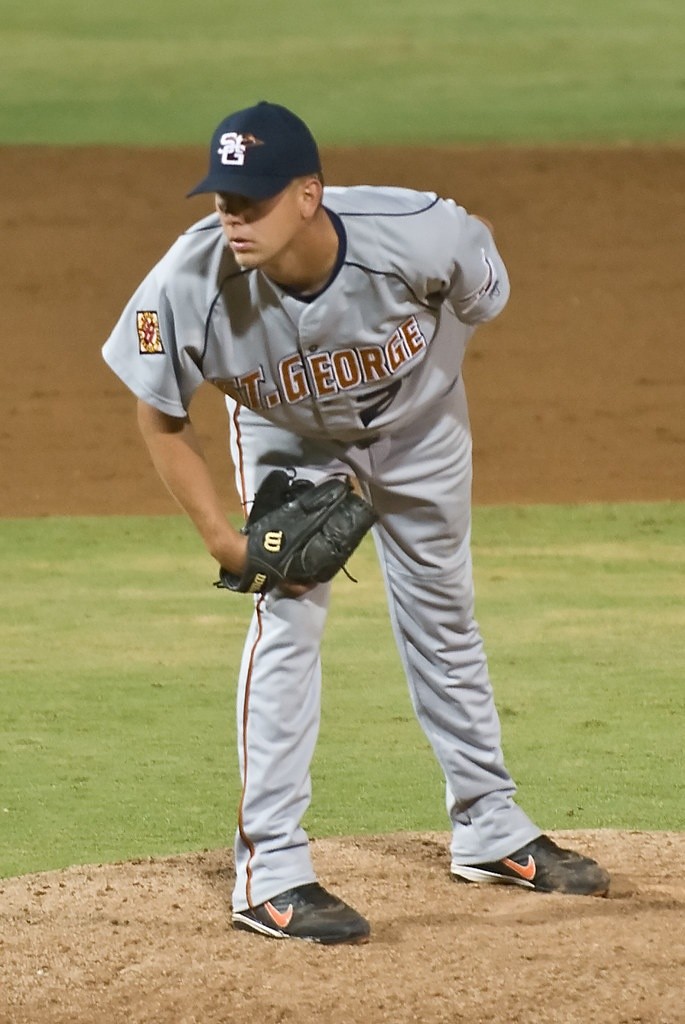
[[104, 101, 612, 947]]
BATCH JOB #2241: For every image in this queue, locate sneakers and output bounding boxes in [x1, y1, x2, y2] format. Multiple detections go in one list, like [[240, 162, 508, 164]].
[[233, 882, 371, 948], [450, 828, 609, 896]]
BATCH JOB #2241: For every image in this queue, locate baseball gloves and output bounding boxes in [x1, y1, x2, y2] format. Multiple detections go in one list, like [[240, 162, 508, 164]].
[[218, 469, 381, 600]]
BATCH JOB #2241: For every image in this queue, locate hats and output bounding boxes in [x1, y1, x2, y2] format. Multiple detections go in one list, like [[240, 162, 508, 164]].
[[185, 101, 323, 202]]
